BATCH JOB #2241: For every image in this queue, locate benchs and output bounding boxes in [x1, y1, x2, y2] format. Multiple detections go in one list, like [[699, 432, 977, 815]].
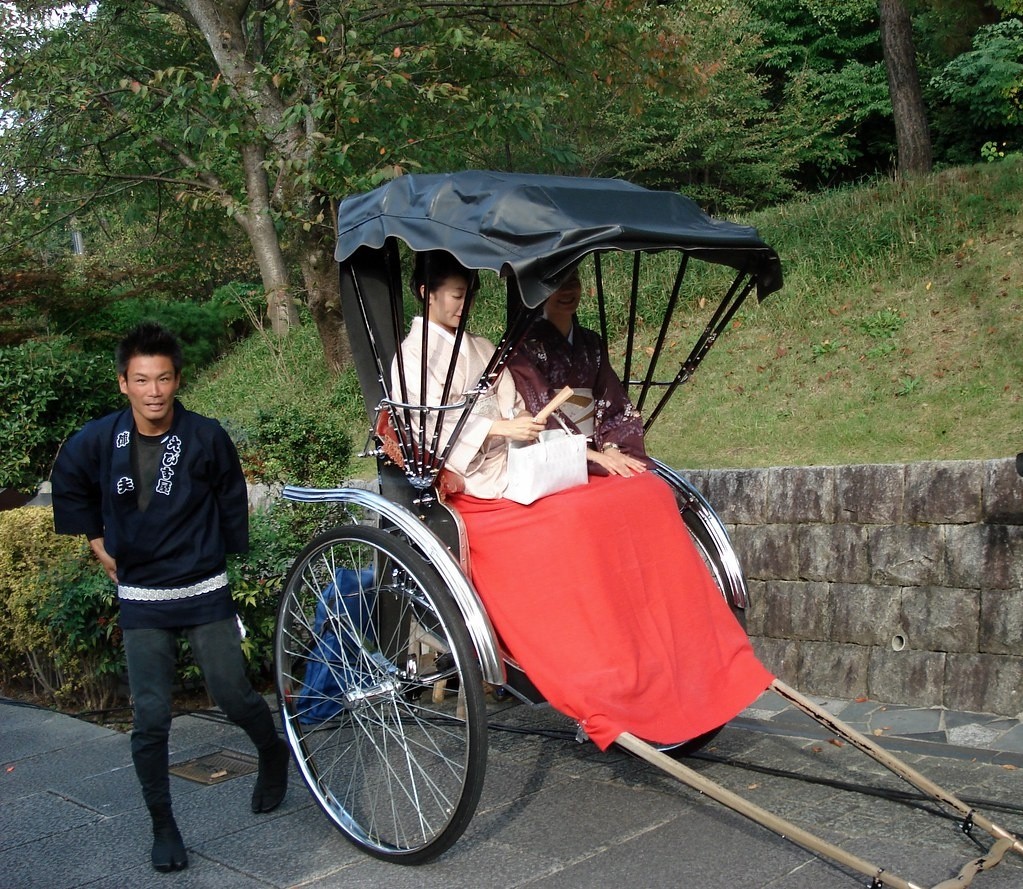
[[375, 403, 734, 603]]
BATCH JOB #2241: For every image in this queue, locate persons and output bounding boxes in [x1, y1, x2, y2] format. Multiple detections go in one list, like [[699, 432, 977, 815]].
[[391, 249, 658, 501], [51, 322, 291, 874]]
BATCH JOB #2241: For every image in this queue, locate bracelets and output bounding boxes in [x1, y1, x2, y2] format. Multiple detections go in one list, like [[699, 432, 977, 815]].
[[602, 442, 620, 451]]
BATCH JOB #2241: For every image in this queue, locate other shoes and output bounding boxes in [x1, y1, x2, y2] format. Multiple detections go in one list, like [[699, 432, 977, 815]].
[[251, 738, 290, 814], [150, 827, 188, 873]]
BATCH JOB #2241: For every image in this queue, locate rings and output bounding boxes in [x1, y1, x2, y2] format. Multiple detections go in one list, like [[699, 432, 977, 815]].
[[533, 417, 537, 423]]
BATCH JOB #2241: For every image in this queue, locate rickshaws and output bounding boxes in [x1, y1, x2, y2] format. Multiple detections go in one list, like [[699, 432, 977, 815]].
[[270, 169, 1022, 889]]
